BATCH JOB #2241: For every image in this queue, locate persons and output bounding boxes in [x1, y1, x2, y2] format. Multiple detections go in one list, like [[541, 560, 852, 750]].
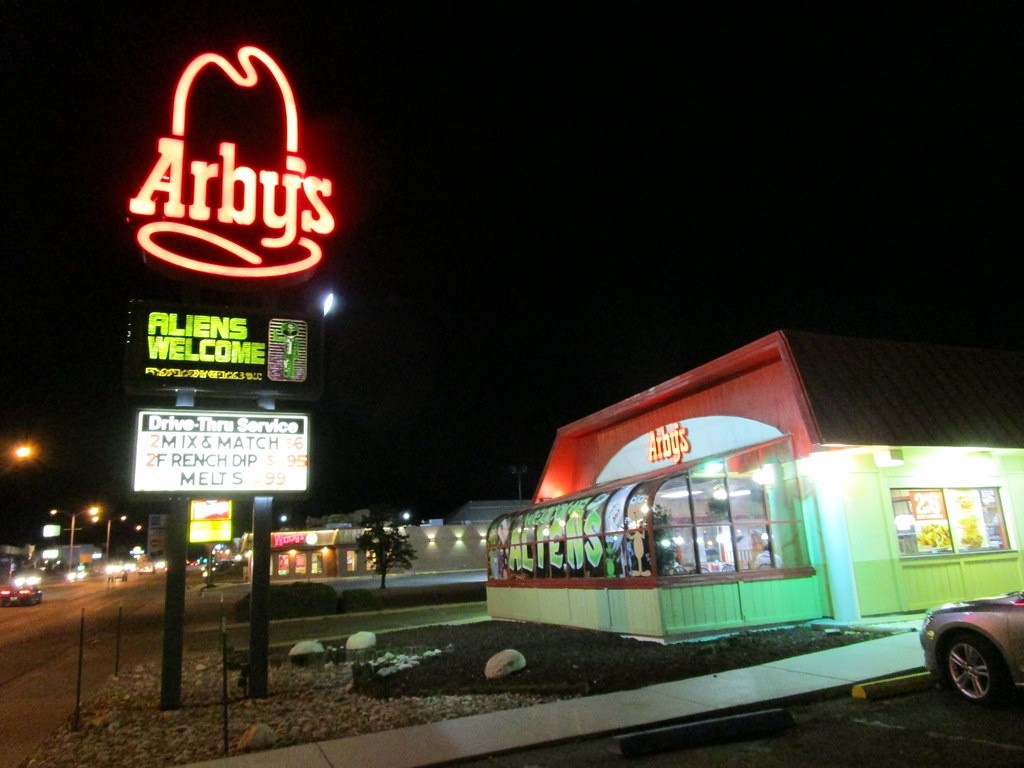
[[755, 545, 782, 568]]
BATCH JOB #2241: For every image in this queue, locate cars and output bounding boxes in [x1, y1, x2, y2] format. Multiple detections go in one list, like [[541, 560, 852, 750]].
[[0, 583, 42, 606], [138, 561, 155, 574], [108, 568, 127, 582], [919, 591, 1024, 705]]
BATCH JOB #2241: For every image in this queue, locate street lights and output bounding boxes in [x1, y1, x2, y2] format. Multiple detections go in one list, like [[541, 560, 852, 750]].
[[51, 505, 98, 576]]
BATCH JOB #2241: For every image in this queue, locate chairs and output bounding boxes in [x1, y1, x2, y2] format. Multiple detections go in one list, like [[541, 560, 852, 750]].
[[672, 563, 713, 574], [722, 564, 734, 572], [757, 563, 771, 568]]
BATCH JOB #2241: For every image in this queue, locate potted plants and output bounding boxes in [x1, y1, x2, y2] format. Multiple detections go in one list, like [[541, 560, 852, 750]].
[[705, 495, 729, 519]]
